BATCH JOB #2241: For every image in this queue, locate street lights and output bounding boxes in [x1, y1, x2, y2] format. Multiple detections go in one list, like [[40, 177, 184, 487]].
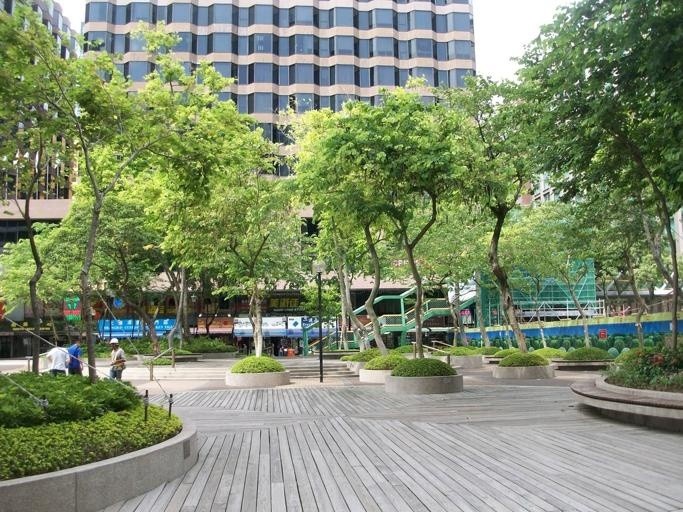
[[190, 293, 196, 337], [310, 261, 325, 382], [281, 315, 289, 338], [105, 288, 116, 341]]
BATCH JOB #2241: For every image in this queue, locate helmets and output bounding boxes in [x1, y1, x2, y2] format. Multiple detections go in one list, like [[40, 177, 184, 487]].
[[110, 338, 118, 344]]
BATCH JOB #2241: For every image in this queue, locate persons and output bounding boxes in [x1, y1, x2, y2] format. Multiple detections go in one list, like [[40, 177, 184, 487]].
[[65, 336, 84, 376], [283, 343, 288, 356], [278, 344, 283, 356], [46, 337, 70, 377], [107, 337, 127, 382]]
